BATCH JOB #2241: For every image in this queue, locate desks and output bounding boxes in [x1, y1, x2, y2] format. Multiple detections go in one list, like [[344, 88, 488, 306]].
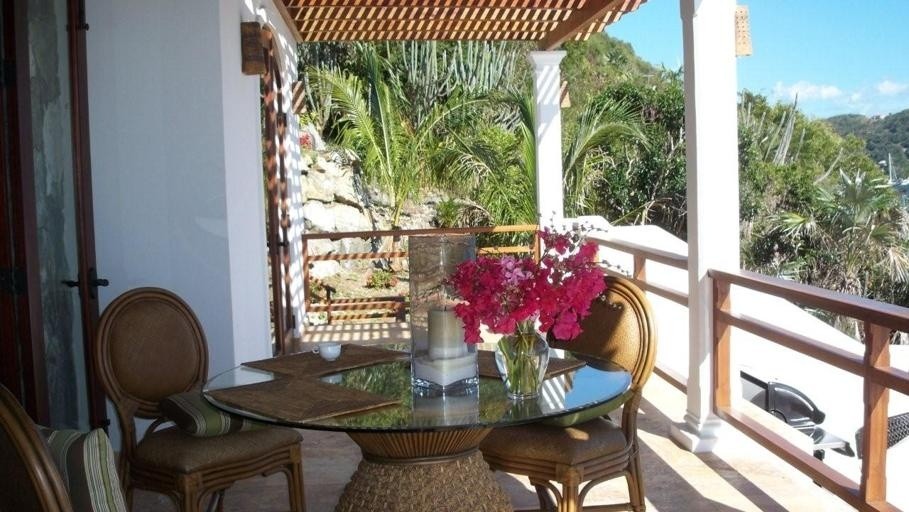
[[205, 338, 634, 511]]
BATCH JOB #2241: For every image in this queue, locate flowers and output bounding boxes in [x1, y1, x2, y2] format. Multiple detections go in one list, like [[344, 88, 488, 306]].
[[449, 209, 611, 392]]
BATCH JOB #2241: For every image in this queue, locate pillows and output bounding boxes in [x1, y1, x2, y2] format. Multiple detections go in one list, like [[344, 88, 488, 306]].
[[32, 424, 132, 512], [158, 391, 277, 437]]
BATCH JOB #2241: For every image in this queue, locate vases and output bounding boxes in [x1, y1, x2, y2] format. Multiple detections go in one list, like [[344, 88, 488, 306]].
[[494, 326, 549, 399]]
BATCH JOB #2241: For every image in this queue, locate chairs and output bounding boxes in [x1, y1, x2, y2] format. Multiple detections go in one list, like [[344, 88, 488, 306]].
[[1, 385, 72, 512], [94, 286, 307, 512], [472, 273, 659, 512]]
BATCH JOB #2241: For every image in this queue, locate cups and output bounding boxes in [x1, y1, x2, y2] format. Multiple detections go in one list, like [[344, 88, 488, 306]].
[[312, 343, 341, 362], [408, 234, 479, 393]]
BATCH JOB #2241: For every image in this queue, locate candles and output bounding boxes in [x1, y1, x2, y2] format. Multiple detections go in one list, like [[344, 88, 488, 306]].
[[429, 303, 467, 360]]
